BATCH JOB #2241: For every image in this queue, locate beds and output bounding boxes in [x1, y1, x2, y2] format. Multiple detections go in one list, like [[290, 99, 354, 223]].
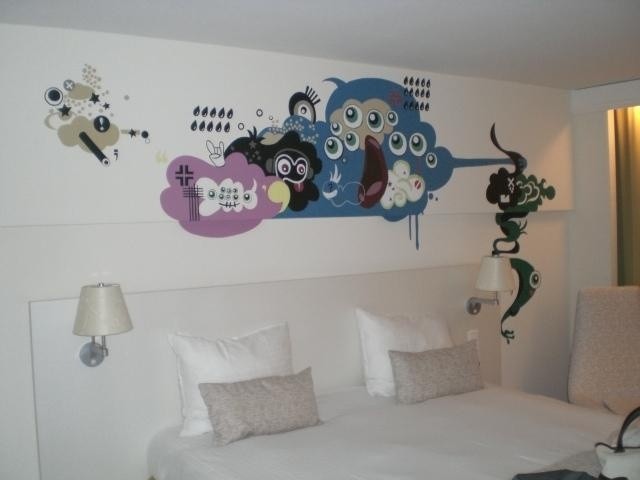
[[148, 381, 639, 480]]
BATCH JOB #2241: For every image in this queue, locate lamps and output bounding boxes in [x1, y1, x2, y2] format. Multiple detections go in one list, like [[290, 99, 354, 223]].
[[73, 285, 134, 366], [466, 256, 516, 316]]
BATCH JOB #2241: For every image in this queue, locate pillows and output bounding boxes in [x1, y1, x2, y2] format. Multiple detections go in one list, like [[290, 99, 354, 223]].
[[353, 305, 456, 400], [165, 321, 294, 437], [197, 366, 321, 443], [387, 339, 485, 404]]
